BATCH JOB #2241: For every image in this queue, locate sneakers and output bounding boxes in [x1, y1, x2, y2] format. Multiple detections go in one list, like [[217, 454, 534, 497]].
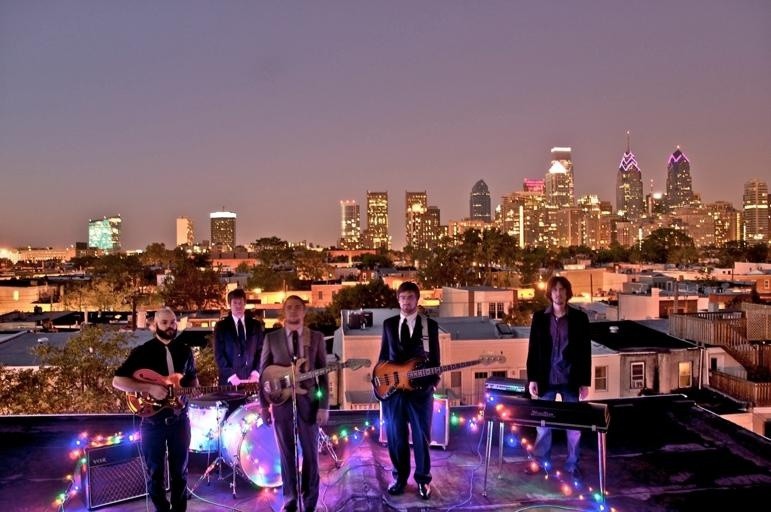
[[573, 466, 589, 475], [524, 466, 544, 474]]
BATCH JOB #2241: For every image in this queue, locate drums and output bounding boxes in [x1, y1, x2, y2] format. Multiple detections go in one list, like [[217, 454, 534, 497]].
[[188, 390, 249, 454], [218, 401, 304, 489]]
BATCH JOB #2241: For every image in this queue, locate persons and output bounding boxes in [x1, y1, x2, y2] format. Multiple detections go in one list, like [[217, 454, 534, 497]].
[[214, 289, 263, 385], [378, 281, 440, 500], [526, 276, 592, 481], [112, 309, 199, 512], [258, 295, 329, 512]]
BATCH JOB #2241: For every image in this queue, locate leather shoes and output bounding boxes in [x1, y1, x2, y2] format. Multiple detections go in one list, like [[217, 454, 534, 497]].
[[388, 478, 407, 494], [418, 482, 432, 499]]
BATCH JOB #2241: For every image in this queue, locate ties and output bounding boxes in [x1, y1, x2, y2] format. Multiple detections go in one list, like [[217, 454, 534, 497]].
[[237, 318, 245, 346], [401, 318, 410, 342], [292, 330, 299, 356], [164, 347, 174, 375]]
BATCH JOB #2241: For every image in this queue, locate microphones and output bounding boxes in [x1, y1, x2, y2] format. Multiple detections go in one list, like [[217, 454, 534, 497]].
[[291, 353, 298, 365]]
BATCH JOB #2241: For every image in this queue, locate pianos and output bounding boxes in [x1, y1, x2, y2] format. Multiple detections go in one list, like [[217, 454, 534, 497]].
[[485, 394, 611, 432]]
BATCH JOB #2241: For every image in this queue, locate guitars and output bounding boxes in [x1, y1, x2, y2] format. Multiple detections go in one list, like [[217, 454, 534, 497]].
[[259, 357, 372, 405], [372, 350, 507, 401], [126, 368, 263, 417]]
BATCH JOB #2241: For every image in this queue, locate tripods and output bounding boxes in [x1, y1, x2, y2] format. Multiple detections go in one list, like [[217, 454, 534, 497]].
[[186, 403, 252, 501]]
